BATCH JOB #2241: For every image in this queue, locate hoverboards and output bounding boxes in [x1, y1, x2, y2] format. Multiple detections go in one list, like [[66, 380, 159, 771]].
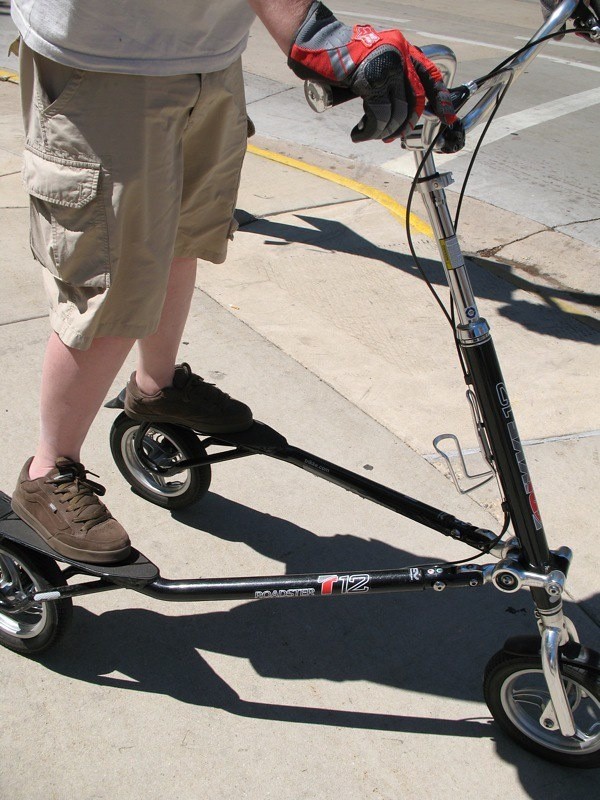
[[0, 0, 599, 773]]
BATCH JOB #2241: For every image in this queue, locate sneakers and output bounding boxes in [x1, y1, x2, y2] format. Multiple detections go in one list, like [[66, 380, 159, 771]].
[[124, 362, 253, 433], [10, 457, 131, 563]]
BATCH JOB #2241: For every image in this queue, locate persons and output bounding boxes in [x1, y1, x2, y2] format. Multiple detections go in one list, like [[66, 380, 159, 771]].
[[10, 0, 600, 564]]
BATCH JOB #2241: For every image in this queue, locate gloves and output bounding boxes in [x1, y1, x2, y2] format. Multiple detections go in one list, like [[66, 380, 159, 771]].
[[538, 0, 600, 43], [287, 1, 456, 143]]
